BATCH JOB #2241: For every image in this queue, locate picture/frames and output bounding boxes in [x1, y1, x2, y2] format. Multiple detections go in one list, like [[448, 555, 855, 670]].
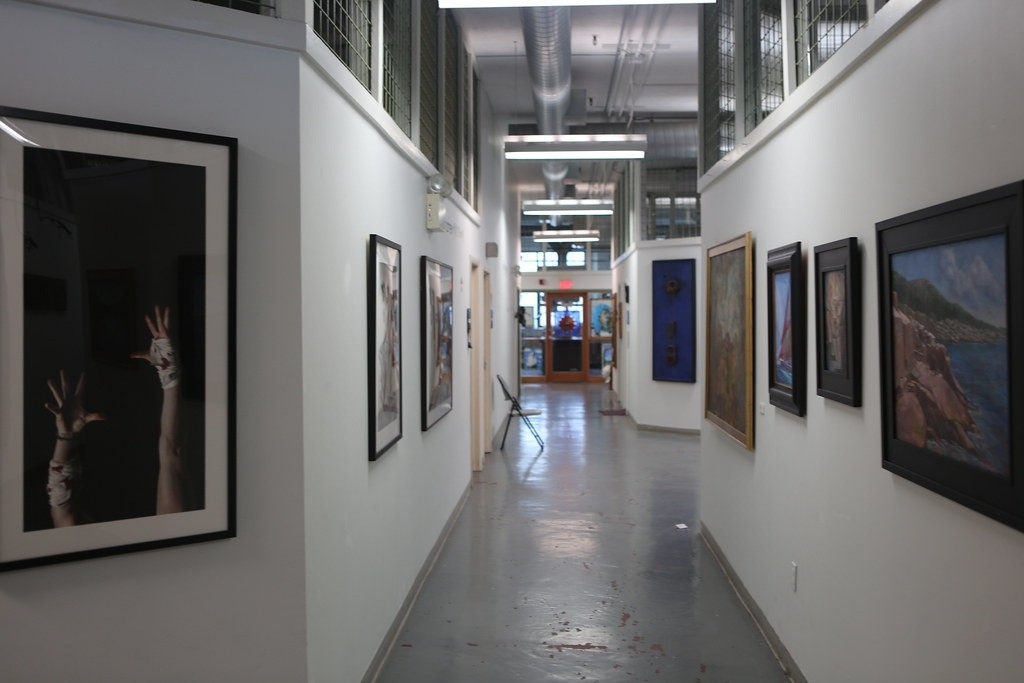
[[368, 233, 404, 462], [652, 259, 697, 382], [0, 106, 238, 569], [704, 232, 755, 449], [419, 255, 454, 432], [766, 241, 808, 416], [873, 179, 1024, 533], [815, 236, 862, 406]]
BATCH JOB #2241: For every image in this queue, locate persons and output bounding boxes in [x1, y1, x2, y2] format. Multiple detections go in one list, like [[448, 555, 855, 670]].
[[44, 304, 186, 529]]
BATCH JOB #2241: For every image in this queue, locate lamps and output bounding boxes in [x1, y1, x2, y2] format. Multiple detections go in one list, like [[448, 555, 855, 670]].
[[427, 175, 452, 198]]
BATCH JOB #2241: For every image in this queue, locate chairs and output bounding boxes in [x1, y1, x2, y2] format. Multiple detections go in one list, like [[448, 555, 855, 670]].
[[494, 375, 544, 451]]
